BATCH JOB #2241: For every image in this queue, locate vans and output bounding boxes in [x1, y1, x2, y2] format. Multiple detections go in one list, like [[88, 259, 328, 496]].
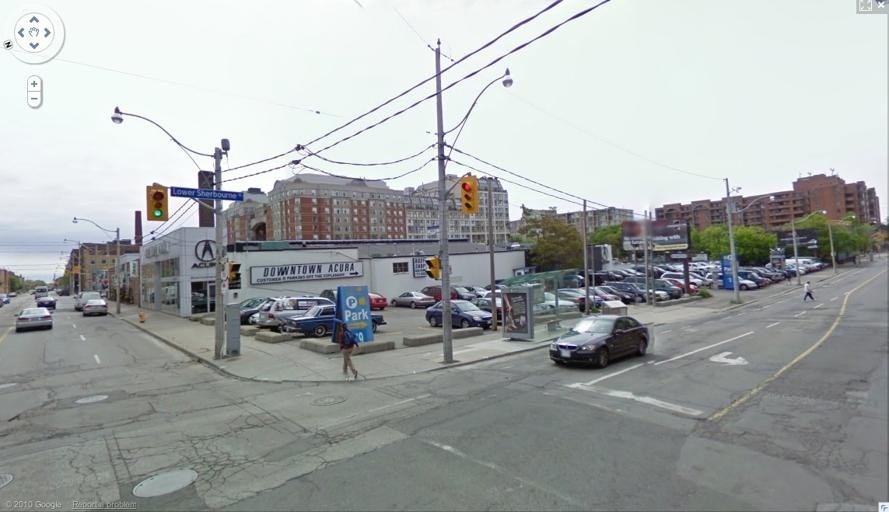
[[34, 286, 48, 300], [191, 296, 215, 311], [259, 297, 337, 332]]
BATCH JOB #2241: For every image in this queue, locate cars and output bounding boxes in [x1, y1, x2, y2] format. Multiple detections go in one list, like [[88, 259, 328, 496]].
[[98, 290, 106, 297], [287, 305, 386, 336], [421, 287, 458, 301], [47, 288, 54, 291], [450, 286, 476, 301], [0, 297, 3, 308], [320, 289, 338, 302], [151, 288, 154, 302], [83, 299, 108, 315], [391, 292, 434, 308], [225, 298, 257, 320], [369, 292, 388, 309], [425, 298, 493, 330], [13, 307, 54, 330], [248, 312, 259, 324], [29, 288, 35, 295], [162, 285, 176, 304], [240, 299, 266, 324], [549, 314, 649, 367], [6, 292, 17, 297], [471, 297, 502, 322], [55, 288, 70, 296], [192, 292, 200, 295], [37, 297, 58, 310], [74, 292, 100, 310], [0, 293, 10, 304]]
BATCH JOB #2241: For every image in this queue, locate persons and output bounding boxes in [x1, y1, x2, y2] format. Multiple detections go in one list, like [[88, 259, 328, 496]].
[[803, 279, 817, 302], [337, 321, 362, 379]]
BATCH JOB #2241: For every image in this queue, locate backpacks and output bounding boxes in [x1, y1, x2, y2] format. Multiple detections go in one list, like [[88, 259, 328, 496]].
[[344, 331, 357, 345]]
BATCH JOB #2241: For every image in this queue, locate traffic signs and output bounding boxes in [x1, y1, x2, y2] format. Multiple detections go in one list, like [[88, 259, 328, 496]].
[[168, 186, 243, 201]]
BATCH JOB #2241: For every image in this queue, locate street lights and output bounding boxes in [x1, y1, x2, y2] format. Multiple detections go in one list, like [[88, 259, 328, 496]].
[[724, 178, 775, 304], [111, 106, 231, 359], [71, 216, 121, 315], [53, 238, 97, 296], [790, 209, 827, 286], [856, 218, 878, 264], [827, 215, 855, 274], [431, 38, 514, 363]]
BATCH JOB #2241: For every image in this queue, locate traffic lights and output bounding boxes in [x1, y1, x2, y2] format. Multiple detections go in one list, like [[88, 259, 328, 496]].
[[459, 176, 480, 215], [146, 183, 170, 222], [424, 256, 440, 280], [227, 261, 241, 284]]
